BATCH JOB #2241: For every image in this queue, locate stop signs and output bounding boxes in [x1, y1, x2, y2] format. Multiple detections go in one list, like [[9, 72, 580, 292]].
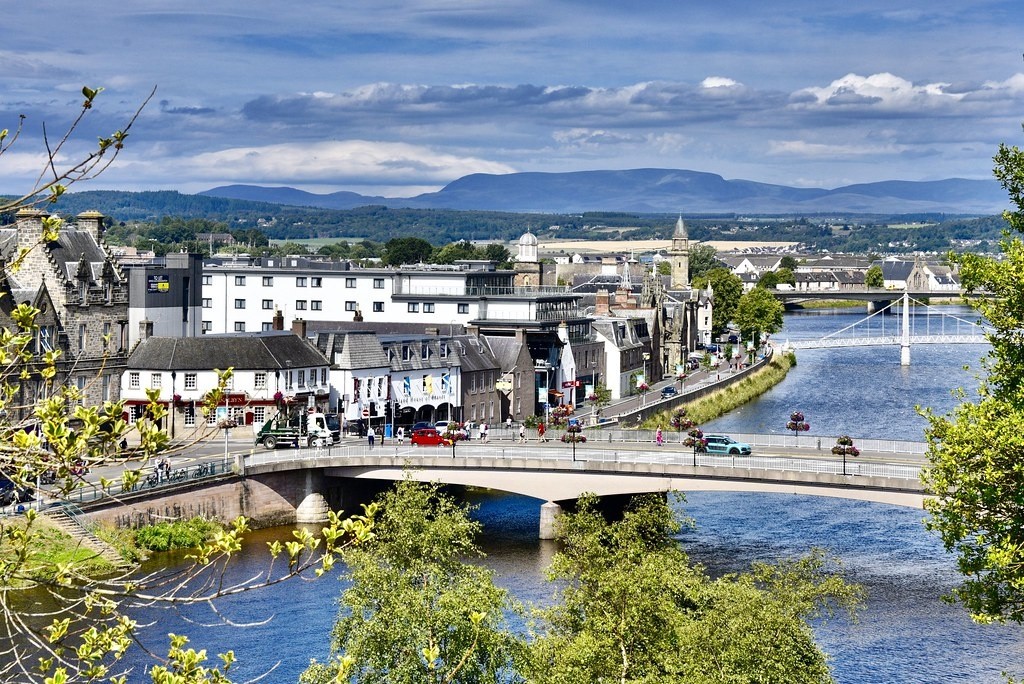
[[308, 407, 314, 414], [363, 409, 369, 416]]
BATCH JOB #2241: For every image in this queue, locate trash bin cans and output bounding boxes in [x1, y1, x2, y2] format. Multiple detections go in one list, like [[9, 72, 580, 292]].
[[386, 424, 392, 438], [570, 420, 578, 425], [17, 505, 24, 511]]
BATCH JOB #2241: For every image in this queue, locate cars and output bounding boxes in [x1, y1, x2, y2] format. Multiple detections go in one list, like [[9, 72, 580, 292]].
[[0, 478, 34, 506], [729, 335, 738, 343], [661, 386, 678, 399], [706, 344, 720, 353], [408, 421, 467, 448]]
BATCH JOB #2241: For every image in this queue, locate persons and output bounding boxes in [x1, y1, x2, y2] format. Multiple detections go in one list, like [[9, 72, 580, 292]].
[[396, 424, 405, 445], [367, 426, 375, 450], [505, 416, 513, 430], [537, 422, 547, 443], [478, 421, 488, 444], [656, 424, 664, 446], [463, 419, 471, 432], [517, 423, 526, 444]]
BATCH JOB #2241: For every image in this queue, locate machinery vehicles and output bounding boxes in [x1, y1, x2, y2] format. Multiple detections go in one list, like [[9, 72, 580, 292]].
[[254, 403, 341, 450]]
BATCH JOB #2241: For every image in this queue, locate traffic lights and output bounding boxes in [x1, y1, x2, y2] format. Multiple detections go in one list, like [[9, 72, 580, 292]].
[[394, 402, 400, 417], [384, 402, 390, 417]]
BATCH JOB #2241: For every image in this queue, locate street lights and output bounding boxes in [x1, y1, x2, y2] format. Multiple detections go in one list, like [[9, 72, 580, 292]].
[[224, 389, 231, 471], [716, 338, 720, 380], [590, 362, 597, 395], [641, 353, 650, 407], [682, 346, 686, 393]]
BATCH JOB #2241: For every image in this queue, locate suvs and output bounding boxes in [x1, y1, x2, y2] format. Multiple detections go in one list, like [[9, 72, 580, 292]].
[[694, 434, 751, 455]]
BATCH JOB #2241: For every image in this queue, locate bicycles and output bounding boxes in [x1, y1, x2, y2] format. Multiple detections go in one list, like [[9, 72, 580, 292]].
[[192, 462, 208, 479], [139, 468, 158, 490], [168, 469, 186, 484]]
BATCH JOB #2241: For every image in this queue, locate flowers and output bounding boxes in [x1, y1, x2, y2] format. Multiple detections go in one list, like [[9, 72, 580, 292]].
[[561, 425, 586, 443], [832, 436, 859, 456], [682, 427, 708, 447], [786, 411, 809, 431], [669, 409, 691, 429], [549, 409, 566, 426], [442, 422, 467, 441]]
